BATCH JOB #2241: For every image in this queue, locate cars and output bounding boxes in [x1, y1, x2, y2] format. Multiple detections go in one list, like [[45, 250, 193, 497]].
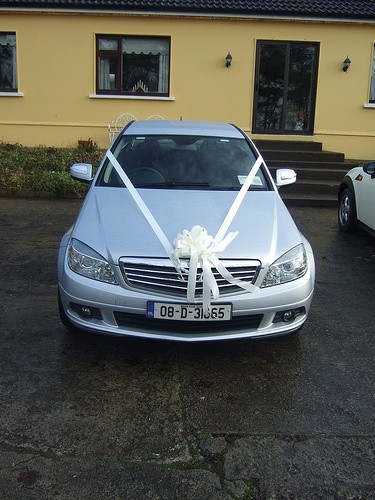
[[337, 161, 375, 237], [55, 119, 316, 346]]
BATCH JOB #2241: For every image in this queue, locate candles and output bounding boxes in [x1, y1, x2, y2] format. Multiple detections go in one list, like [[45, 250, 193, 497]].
[[133, 80, 148, 92]]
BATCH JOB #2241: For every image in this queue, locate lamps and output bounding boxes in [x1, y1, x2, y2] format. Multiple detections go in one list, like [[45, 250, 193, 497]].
[[343, 56, 351, 72], [225, 52, 233, 68]]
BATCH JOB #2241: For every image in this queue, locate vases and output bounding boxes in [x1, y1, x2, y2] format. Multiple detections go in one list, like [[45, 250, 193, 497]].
[[78, 140, 90, 148]]
